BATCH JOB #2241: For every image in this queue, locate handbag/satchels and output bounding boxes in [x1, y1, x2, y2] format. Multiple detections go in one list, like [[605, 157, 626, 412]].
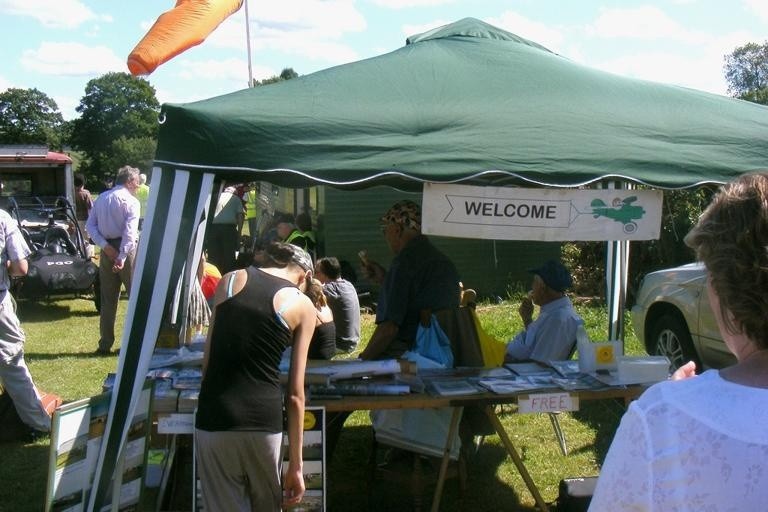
[[533, 476, 599, 512], [412, 314, 453, 369]]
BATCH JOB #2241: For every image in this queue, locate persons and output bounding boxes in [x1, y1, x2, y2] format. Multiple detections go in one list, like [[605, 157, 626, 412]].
[[1, 208, 52, 433], [132, 173, 150, 232], [96, 175, 113, 196], [583, 171, 768, 512], [324, 198, 461, 465], [74, 173, 98, 260], [504, 258, 581, 365], [182, 184, 362, 361], [192, 241, 317, 511], [84, 164, 142, 355]]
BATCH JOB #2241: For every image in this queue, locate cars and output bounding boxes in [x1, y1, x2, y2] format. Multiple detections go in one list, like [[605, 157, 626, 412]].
[[631, 261, 737, 375]]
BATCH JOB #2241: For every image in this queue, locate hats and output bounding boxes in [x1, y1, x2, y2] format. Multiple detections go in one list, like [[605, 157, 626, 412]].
[[526, 260, 571, 291], [381, 199, 420, 231], [288, 243, 314, 283]]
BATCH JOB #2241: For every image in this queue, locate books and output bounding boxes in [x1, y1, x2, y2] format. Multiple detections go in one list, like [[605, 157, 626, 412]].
[[99, 336, 330, 511], [331, 340, 672, 418]]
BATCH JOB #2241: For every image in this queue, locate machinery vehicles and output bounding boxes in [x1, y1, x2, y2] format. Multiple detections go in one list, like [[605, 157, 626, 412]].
[[1, 144, 100, 313]]
